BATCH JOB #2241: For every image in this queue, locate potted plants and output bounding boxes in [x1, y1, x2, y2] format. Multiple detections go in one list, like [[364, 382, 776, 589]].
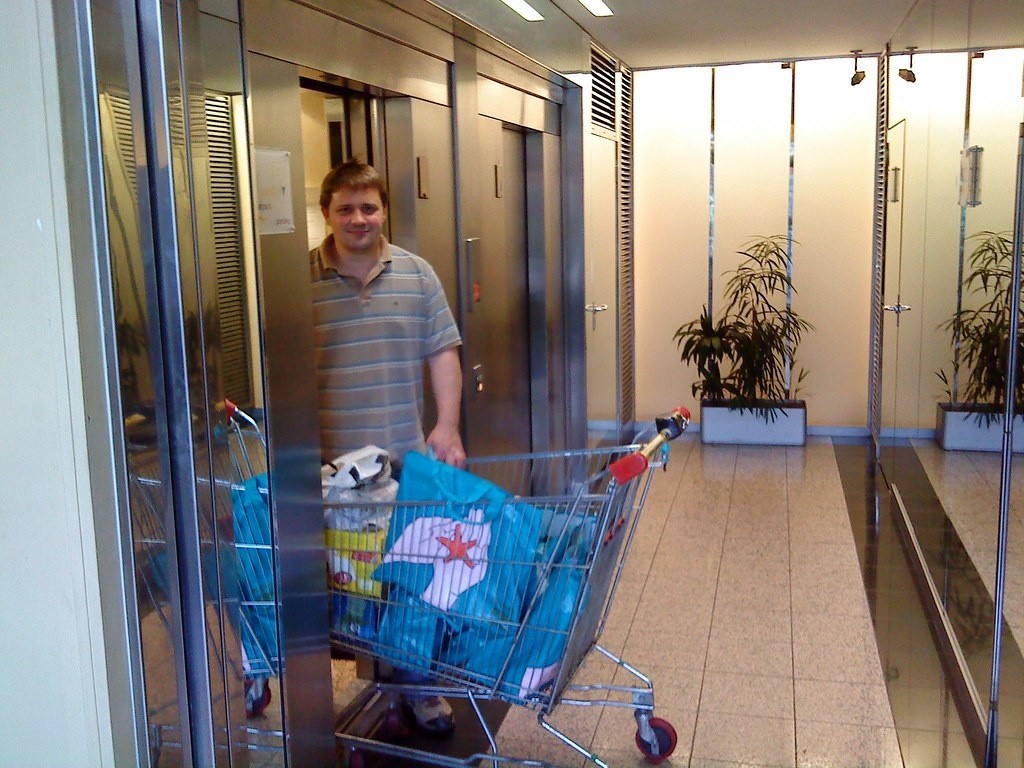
[[934, 228, 1024, 452], [672, 234, 817, 445]]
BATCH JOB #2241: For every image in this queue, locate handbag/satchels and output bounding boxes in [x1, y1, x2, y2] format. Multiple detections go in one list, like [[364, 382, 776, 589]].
[[226, 444, 600, 704]]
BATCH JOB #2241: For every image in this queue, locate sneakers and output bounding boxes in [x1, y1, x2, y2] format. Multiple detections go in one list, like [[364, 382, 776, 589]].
[[402, 693, 457, 732]]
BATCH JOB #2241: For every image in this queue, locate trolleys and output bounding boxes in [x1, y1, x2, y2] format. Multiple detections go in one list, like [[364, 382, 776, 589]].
[[125, 388, 690, 768]]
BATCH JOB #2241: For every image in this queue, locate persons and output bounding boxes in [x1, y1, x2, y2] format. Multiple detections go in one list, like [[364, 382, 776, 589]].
[[309, 151, 463, 726]]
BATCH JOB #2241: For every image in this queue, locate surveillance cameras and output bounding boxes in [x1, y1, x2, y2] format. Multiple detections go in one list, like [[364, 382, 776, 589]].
[[898, 69, 916, 83], [851, 71, 866, 86]]
